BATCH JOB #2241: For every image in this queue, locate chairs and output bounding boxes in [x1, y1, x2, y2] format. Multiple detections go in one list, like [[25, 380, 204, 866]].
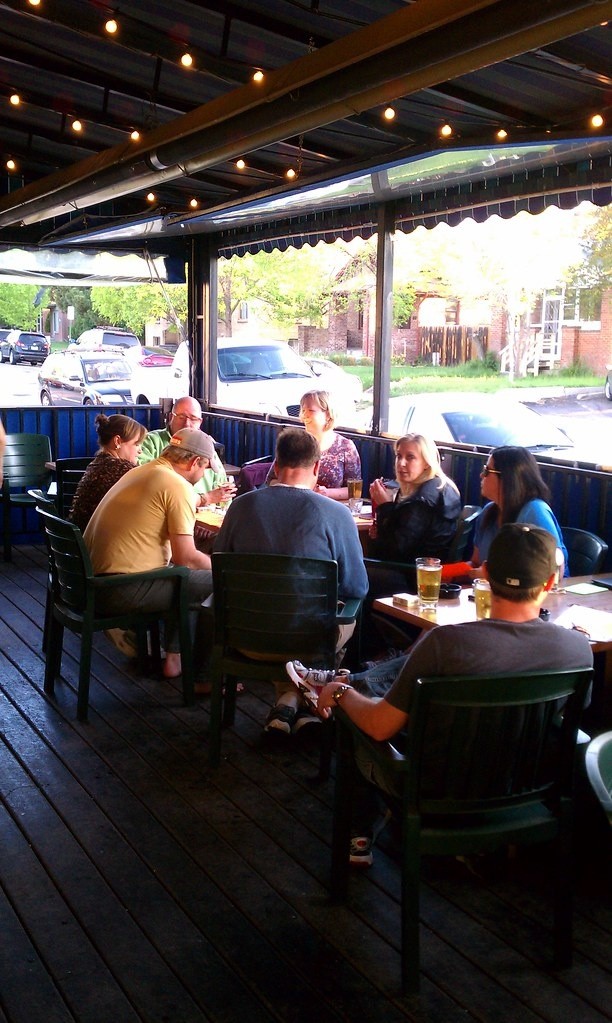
[[56, 457, 96, 520], [247, 356, 271, 374], [584, 730, 612, 825], [201, 552, 364, 771], [87, 366, 100, 380], [327, 665, 596, 995], [363, 505, 484, 655], [106, 365, 119, 378], [561, 527, 608, 578], [0, 432, 55, 561], [36, 504, 197, 721], [27, 488, 162, 672], [219, 356, 239, 375]]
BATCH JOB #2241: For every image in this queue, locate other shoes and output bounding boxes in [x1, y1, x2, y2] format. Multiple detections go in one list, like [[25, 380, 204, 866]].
[[104, 628, 140, 657], [195, 681, 247, 696]]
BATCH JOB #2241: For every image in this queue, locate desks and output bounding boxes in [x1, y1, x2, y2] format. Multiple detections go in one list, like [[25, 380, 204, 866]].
[[376, 571, 612, 654], [194, 498, 375, 544], [45, 461, 243, 476]]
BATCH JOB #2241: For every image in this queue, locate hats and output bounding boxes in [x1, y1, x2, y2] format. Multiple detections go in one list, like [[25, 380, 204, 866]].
[[486, 522, 565, 589], [170, 427, 219, 473]]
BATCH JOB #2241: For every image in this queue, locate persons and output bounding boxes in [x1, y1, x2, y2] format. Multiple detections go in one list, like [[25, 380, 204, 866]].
[[134, 396, 233, 515], [285, 522, 594, 869], [465, 445, 571, 583], [0, 417, 7, 489], [68, 414, 240, 659], [211, 427, 369, 744], [82, 427, 247, 698], [266, 389, 362, 501], [360, 433, 461, 632]]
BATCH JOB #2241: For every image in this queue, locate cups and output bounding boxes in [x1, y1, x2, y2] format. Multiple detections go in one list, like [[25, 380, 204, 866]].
[[219, 475, 233, 511], [549, 551, 559, 591], [415, 558, 443, 608], [473, 577, 491, 621], [349, 498, 363, 516], [347, 478, 363, 499]]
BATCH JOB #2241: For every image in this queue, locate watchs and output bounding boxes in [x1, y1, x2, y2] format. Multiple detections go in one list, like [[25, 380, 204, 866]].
[[331, 685, 354, 707]]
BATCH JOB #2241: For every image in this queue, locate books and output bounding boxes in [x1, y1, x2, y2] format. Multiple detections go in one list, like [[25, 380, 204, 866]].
[[359, 505, 373, 520], [591, 576, 612, 590]]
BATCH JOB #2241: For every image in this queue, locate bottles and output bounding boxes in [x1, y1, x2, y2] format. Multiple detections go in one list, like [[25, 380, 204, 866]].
[[371, 483, 387, 536]]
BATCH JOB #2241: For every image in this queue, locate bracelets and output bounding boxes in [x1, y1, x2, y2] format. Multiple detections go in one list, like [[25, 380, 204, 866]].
[[197, 492, 206, 507]]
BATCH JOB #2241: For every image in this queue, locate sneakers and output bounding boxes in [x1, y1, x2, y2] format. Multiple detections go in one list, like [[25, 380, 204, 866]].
[[350, 837, 375, 867], [264, 702, 323, 734], [286, 658, 343, 721]]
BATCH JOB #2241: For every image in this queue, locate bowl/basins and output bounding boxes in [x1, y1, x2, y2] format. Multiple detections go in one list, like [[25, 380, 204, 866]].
[[197, 507, 211, 513]]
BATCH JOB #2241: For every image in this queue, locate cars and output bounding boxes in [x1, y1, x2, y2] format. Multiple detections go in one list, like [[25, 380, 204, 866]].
[[302, 356, 366, 406], [604, 364, 612, 404], [125, 345, 175, 376], [343, 390, 577, 460]]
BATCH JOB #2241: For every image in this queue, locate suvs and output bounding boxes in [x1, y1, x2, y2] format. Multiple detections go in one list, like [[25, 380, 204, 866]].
[[169, 335, 329, 422], [68, 326, 142, 354], [38, 349, 136, 405], [0, 327, 50, 366]]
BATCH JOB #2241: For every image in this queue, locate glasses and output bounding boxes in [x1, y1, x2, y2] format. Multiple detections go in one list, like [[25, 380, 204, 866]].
[[482, 464, 501, 477], [172, 411, 203, 424]]
